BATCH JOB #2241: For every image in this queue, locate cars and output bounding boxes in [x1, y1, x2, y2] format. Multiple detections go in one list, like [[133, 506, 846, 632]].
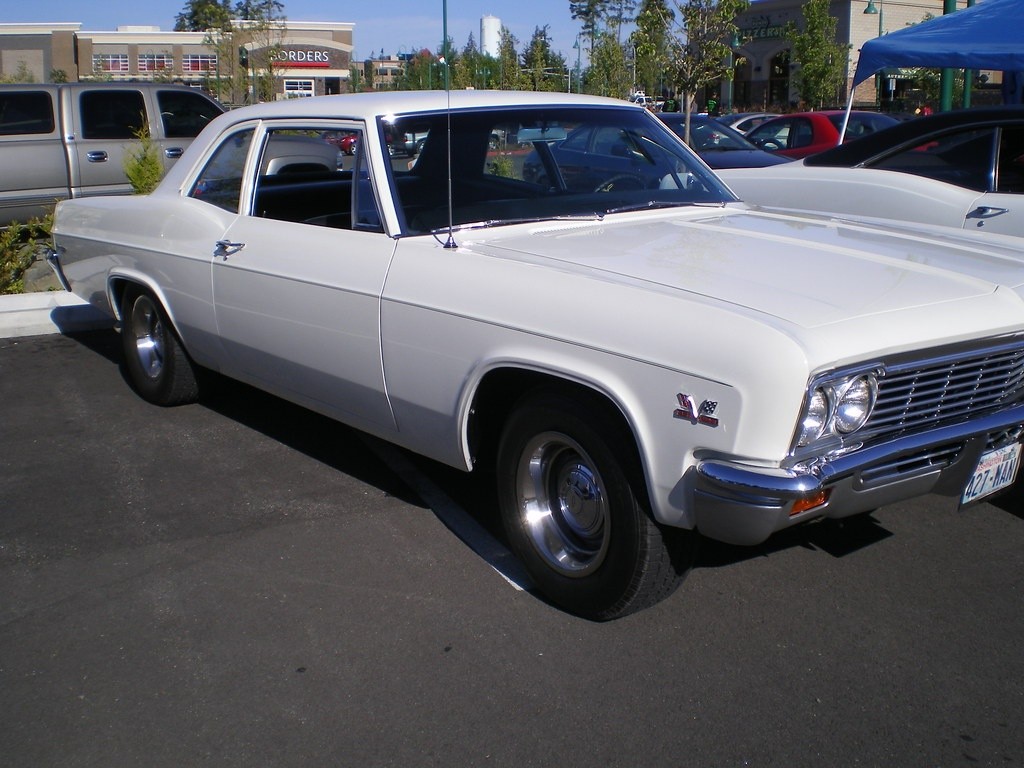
[[521, 111, 797, 189], [46, 77, 1024, 624], [314, 88, 713, 163], [658, 106, 1024, 241], [722, 109, 940, 160]]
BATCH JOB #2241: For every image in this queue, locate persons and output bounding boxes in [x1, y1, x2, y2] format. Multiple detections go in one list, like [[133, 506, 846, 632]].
[[708, 91, 722, 117], [662, 92, 680, 112]]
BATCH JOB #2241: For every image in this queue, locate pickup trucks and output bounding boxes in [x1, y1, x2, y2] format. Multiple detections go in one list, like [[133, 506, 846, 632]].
[[1, 80, 344, 229]]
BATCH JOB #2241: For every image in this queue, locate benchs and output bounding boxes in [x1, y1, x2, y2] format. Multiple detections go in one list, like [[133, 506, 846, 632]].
[[203, 173, 421, 224]]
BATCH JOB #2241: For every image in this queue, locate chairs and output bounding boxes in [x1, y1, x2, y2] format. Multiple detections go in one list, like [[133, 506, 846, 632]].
[[873, 153, 989, 192]]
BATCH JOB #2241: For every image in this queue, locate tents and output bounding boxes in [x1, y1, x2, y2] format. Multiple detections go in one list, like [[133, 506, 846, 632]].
[[837, 0, 1024, 145]]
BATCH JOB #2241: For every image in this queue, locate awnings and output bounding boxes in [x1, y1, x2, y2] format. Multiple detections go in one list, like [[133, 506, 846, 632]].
[[882, 66, 937, 79]]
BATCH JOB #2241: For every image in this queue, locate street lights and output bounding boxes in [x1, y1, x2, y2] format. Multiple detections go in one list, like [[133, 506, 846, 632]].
[[727, 32, 740, 116], [863, 0, 883, 113]]
